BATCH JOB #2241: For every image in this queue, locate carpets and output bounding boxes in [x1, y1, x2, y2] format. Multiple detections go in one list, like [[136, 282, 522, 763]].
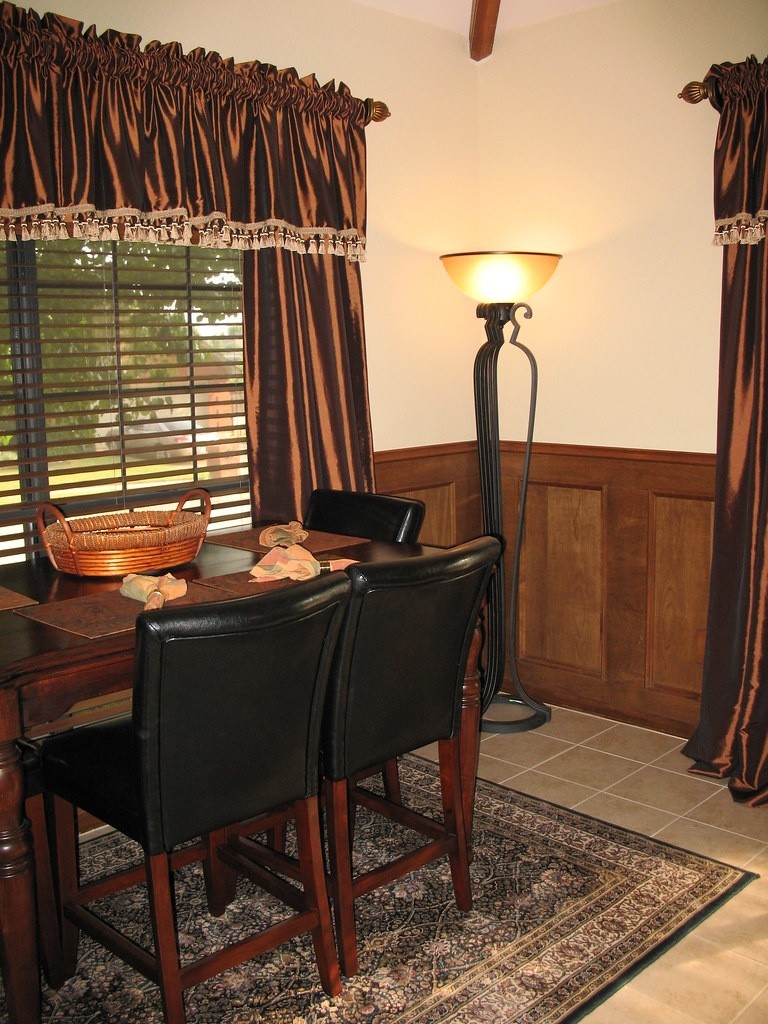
[[1, 750, 761, 1023]]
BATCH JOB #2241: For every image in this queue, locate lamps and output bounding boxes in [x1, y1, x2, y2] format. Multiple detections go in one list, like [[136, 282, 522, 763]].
[[440, 249, 567, 734]]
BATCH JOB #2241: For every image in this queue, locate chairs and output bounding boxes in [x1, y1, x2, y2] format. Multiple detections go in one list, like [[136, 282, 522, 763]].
[[0, 485, 508, 1023]]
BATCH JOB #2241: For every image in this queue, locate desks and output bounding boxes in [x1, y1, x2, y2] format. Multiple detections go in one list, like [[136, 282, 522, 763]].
[[0, 519, 475, 1023]]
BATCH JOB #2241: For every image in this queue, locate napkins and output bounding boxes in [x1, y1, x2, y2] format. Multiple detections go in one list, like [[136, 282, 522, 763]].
[[249, 545, 365, 589], [118, 572, 189, 614], [258, 520, 308, 547]]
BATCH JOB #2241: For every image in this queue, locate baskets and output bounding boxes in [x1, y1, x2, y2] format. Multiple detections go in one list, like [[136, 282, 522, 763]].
[[35, 489, 211, 576]]
